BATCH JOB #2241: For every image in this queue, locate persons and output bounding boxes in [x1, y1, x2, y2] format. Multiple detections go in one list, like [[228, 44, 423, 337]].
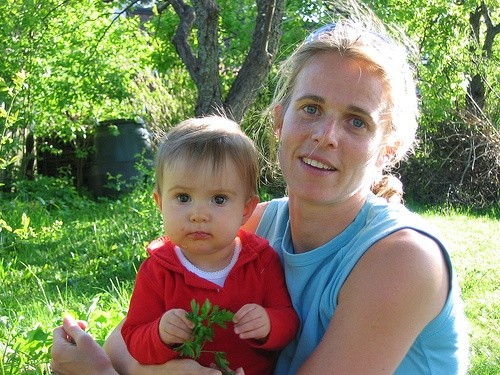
[[119, 105, 299, 375], [50, 25, 472, 375]]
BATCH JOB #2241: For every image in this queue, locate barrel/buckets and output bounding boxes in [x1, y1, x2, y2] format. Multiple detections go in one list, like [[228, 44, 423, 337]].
[[90, 119, 154, 202]]
